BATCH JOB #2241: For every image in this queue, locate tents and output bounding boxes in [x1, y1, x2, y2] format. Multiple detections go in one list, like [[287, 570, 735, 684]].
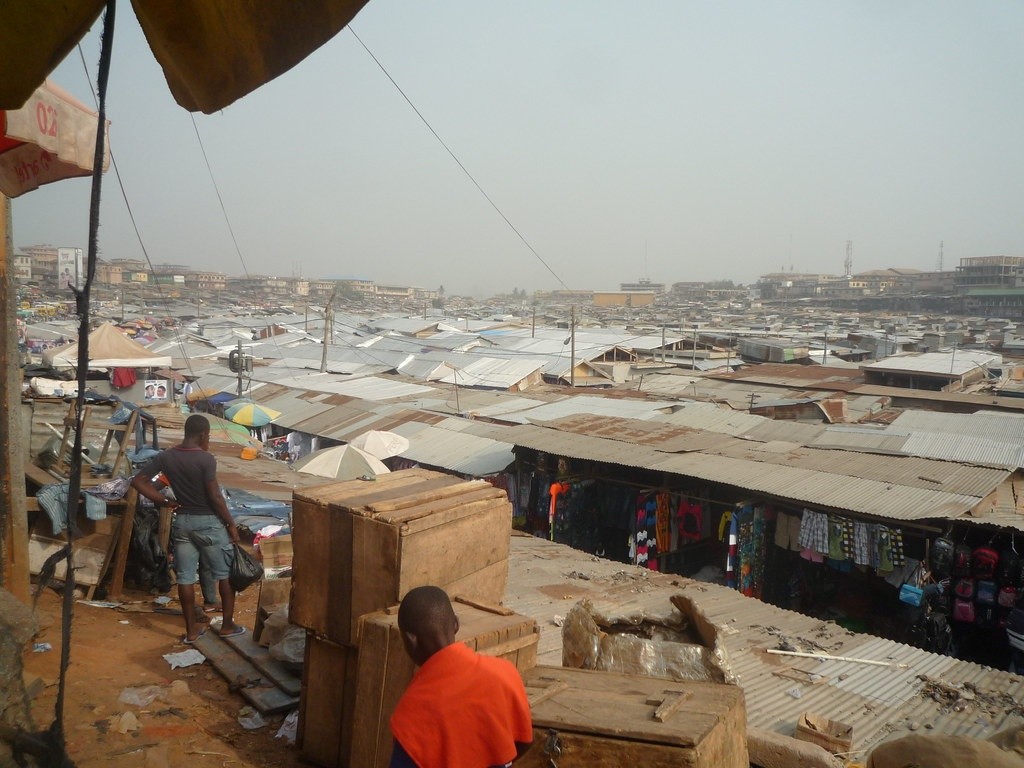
[[41, 319, 178, 405]]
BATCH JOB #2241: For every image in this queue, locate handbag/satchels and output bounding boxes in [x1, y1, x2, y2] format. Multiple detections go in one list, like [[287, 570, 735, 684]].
[[898, 564, 924, 607], [228, 543, 264, 592]]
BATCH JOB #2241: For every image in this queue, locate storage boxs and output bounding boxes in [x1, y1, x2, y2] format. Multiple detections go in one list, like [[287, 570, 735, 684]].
[[287, 466, 751, 768], [792, 711, 854, 763]]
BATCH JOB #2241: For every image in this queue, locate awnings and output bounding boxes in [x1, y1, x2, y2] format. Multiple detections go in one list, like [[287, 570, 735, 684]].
[[0, 77, 112, 199], [384, 418, 1024, 539]]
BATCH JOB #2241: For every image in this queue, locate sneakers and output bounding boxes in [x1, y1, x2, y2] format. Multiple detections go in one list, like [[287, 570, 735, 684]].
[[204, 601, 222, 612]]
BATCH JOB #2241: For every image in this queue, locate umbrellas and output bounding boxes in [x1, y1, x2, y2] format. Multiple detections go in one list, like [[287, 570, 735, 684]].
[[224, 401, 282, 428], [348, 427, 410, 461], [290, 443, 391, 481], [187, 385, 239, 413], [123, 316, 176, 346]]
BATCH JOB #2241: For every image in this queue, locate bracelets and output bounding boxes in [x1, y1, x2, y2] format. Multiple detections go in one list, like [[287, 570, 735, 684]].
[[226, 518, 234, 527], [158, 496, 171, 506]]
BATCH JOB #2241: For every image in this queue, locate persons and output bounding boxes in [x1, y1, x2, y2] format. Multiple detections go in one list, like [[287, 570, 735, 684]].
[[153, 433, 223, 622], [387, 585, 539, 768], [131, 414, 247, 643]]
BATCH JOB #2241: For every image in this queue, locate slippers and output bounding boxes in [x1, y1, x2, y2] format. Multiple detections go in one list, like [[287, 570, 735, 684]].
[[219, 626, 245, 637], [183, 627, 207, 644]]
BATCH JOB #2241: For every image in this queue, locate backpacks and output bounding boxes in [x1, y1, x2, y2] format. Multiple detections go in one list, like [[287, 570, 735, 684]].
[[954, 575, 978, 600], [976, 603, 997, 630], [953, 541, 973, 578], [998, 584, 1016, 608], [930, 537, 955, 577], [952, 597, 975, 623], [909, 610, 962, 659], [976, 578, 1000, 607], [1005, 607, 1024, 651], [972, 545, 1000, 581]]
